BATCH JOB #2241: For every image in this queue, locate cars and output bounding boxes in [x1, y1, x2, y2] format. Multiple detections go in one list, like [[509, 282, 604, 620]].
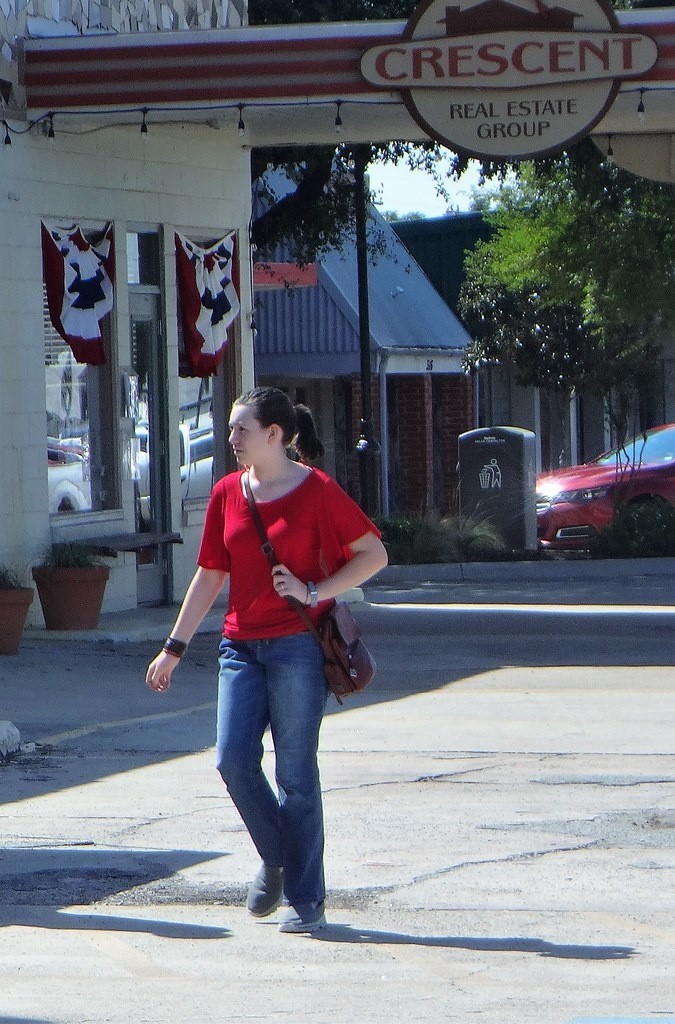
[[47, 412, 213, 533], [537, 423, 674, 558]]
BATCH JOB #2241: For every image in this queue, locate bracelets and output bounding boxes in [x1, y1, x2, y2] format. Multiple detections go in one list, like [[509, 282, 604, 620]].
[[161, 637, 190, 661], [306, 580, 318, 610], [145, 387, 389, 933]]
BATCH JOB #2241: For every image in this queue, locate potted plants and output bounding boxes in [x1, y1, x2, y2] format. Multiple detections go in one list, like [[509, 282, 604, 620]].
[[0, 562, 34, 655], [32, 537, 110, 630]]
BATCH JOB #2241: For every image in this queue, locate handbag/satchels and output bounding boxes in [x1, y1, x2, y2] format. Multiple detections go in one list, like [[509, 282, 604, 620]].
[[317, 599, 377, 705]]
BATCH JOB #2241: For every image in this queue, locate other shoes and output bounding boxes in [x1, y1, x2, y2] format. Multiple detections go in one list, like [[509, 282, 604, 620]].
[[246, 862, 285, 918], [277, 900, 327, 933]]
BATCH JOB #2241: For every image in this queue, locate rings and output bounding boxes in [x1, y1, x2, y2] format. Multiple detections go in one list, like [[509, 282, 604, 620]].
[[279, 584, 284, 590]]
